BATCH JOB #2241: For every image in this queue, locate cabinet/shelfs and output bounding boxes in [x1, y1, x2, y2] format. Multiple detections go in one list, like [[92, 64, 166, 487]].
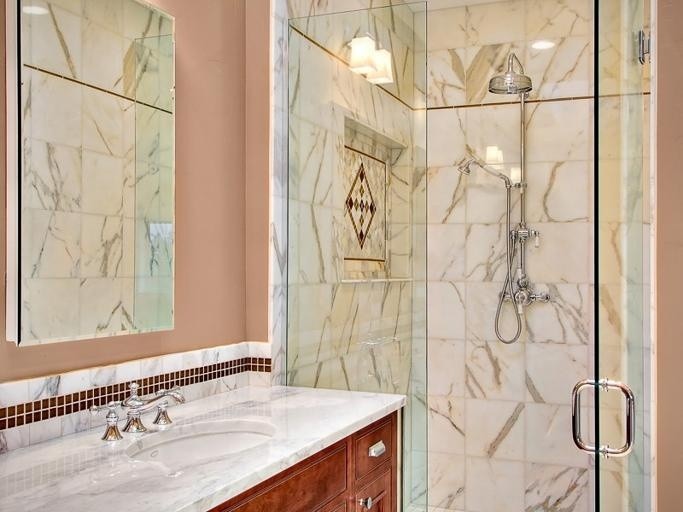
[[207, 411, 396, 511]]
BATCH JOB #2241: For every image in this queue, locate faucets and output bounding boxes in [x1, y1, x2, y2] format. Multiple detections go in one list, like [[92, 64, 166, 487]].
[[29, 375, 61, 399], [119, 383, 186, 432]]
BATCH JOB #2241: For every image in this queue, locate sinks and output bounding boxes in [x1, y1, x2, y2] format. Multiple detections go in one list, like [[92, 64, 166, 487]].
[[125, 419, 275, 462]]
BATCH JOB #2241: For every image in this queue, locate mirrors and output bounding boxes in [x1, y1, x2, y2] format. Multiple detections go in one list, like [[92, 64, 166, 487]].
[[4, 0, 180, 348]]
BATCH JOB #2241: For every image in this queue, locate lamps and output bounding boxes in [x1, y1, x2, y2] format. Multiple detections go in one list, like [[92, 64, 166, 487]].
[[346, 34, 395, 84], [484, 145, 522, 185]]
